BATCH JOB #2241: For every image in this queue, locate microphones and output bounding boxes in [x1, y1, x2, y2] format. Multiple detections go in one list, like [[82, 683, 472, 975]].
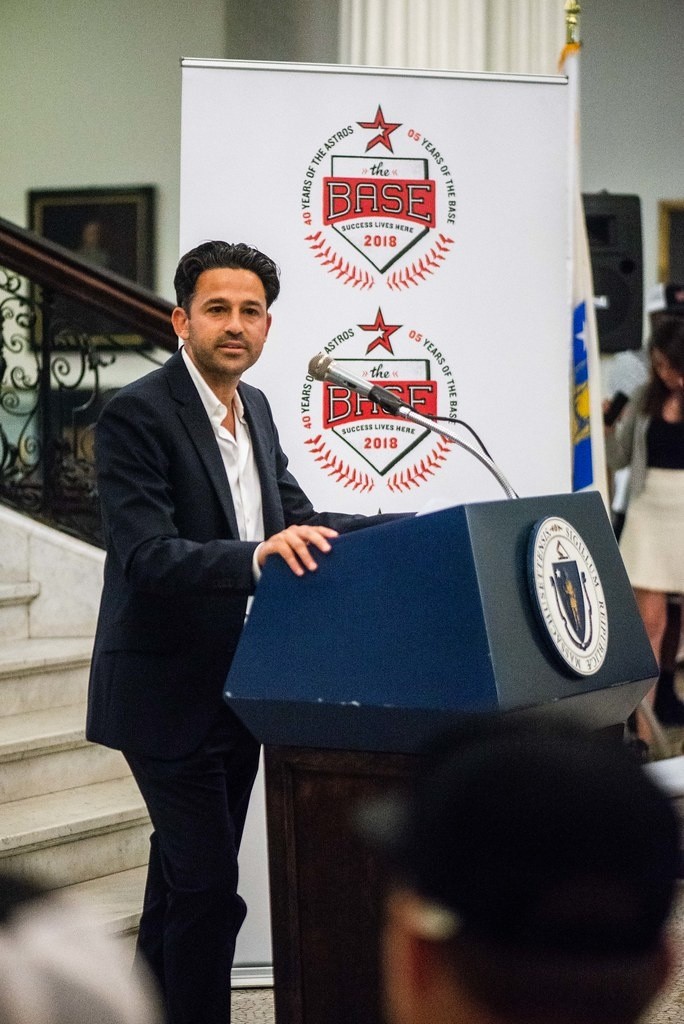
[[308, 355, 518, 500]]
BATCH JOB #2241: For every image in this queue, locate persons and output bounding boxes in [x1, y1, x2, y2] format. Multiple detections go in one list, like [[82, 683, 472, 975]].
[[86, 241, 369, 1024], [601, 292, 684, 753], [383, 711, 684, 1024]]
